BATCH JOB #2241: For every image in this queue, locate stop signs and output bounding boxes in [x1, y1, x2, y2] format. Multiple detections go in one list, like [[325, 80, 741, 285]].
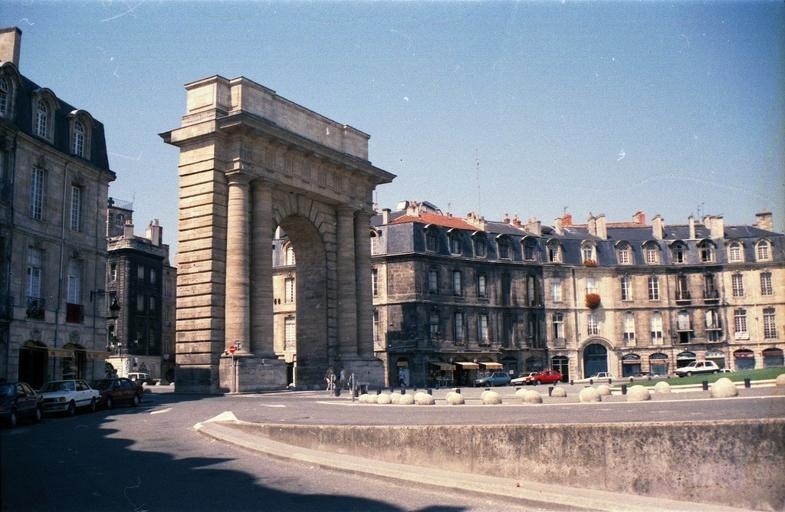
[[229, 346, 235, 353]]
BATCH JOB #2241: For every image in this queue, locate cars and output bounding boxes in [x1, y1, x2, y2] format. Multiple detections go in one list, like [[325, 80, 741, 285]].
[[719, 368, 732, 372], [673, 358, 721, 377], [525, 369, 563, 386], [472, 371, 510, 387], [509, 371, 536, 386], [0, 370, 151, 431]]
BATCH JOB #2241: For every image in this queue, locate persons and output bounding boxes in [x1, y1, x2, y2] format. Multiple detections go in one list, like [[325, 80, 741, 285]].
[[398, 368, 407, 387], [104, 370, 109, 378], [324, 365, 336, 391], [340, 367, 346, 383], [349, 373, 352, 393], [112, 369, 117, 378]]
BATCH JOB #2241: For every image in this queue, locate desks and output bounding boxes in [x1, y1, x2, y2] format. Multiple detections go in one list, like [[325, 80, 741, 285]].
[[437, 378, 451, 388]]
